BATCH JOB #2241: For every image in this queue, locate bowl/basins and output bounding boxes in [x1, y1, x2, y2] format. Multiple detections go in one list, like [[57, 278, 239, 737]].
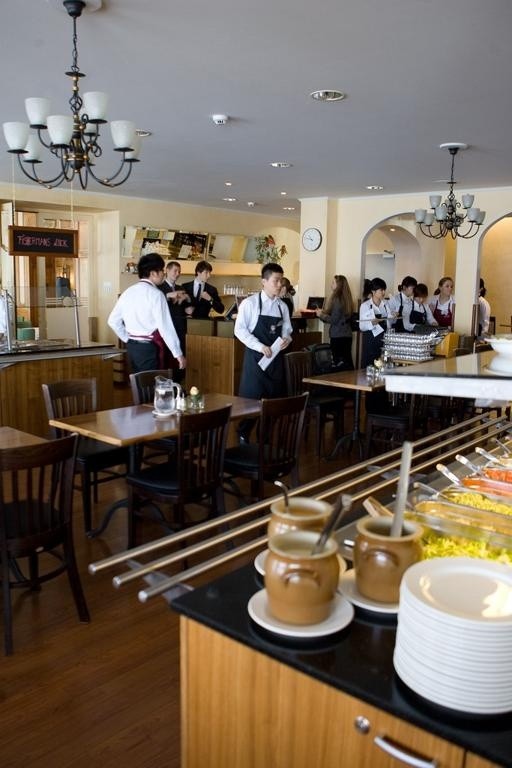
[[484, 338, 512, 355], [483, 355, 512, 377]]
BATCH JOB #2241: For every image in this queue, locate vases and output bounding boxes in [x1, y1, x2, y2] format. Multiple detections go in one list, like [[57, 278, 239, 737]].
[[190, 397, 198, 408]]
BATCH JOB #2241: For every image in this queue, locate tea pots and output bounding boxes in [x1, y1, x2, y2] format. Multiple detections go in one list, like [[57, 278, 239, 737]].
[[154, 375, 183, 413]]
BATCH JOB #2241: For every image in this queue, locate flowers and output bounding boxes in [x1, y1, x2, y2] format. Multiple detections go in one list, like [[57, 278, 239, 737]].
[[190, 385, 200, 397], [254, 234, 288, 266]]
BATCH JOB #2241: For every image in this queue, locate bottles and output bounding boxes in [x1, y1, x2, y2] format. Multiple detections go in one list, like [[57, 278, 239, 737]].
[[263, 497, 425, 625]]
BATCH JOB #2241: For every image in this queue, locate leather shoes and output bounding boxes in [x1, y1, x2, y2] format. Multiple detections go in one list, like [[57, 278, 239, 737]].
[[238, 429, 248, 445]]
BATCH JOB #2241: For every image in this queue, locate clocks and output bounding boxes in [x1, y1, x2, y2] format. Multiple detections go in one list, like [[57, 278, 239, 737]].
[[302, 228, 322, 252]]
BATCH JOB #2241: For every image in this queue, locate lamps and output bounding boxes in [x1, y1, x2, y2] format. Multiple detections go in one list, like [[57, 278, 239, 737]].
[[414, 142, 485, 240], [3, 0, 142, 192]]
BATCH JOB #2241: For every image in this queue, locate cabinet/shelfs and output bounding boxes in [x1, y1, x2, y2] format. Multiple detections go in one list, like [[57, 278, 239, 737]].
[[178, 615, 505, 768]]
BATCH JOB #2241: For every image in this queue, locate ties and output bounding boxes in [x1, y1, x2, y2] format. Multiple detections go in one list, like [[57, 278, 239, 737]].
[[196, 284, 201, 301]]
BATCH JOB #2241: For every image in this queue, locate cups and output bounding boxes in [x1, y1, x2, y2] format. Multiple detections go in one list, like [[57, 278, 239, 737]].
[[187, 394, 205, 409]]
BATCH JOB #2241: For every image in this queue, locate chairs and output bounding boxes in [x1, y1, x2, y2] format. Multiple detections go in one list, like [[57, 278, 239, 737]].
[[1, 431, 95, 647], [226, 391, 312, 539], [184, 316, 502, 462], [130, 367, 215, 454], [124, 400, 235, 582], [39, 377, 130, 532]]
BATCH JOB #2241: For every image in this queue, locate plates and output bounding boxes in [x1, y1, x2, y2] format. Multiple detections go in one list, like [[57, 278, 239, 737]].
[[152, 410, 177, 417], [337, 567, 401, 613], [247, 588, 355, 637], [393, 554, 512, 715], [254, 550, 347, 578]]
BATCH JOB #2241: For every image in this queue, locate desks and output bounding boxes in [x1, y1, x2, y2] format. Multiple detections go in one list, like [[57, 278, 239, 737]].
[[48, 395, 263, 542], [0, 338, 119, 435], [1, 425, 49, 447]]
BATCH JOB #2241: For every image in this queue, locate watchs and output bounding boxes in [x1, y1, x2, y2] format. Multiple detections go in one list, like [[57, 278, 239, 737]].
[[207, 296, 213, 302]]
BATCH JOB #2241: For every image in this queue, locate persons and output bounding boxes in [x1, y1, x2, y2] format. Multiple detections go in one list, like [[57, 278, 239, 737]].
[[107, 252, 187, 405], [232, 278, 296, 355], [315, 274, 356, 401], [181, 260, 225, 320], [157, 262, 192, 398], [234, 262, 296, 447], [359, 276, 490, 420]]
